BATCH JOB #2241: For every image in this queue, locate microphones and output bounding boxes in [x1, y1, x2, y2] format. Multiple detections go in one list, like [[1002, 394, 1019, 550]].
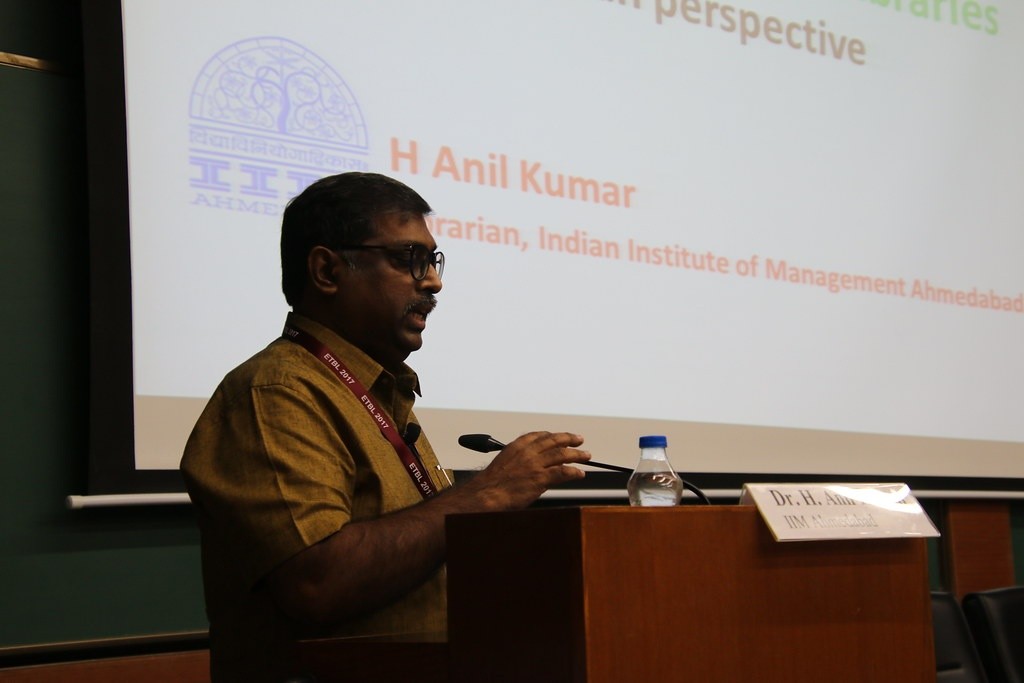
[[403, 422, 421, 443], [458, 434, 711, 504]]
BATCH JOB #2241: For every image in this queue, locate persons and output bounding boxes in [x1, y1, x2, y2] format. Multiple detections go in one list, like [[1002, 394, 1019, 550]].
[[180, 171, 591, 683]]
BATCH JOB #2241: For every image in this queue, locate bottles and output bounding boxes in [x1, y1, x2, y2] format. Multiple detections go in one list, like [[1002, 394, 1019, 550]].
[[627, 436, 683, 506]]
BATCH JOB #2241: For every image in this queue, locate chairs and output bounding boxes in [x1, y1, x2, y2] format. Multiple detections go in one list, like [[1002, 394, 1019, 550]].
[[930, 586, 1024, 683]]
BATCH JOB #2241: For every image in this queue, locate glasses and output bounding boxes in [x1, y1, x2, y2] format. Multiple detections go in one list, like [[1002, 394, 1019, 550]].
[[332, 243, 445, 281]]
